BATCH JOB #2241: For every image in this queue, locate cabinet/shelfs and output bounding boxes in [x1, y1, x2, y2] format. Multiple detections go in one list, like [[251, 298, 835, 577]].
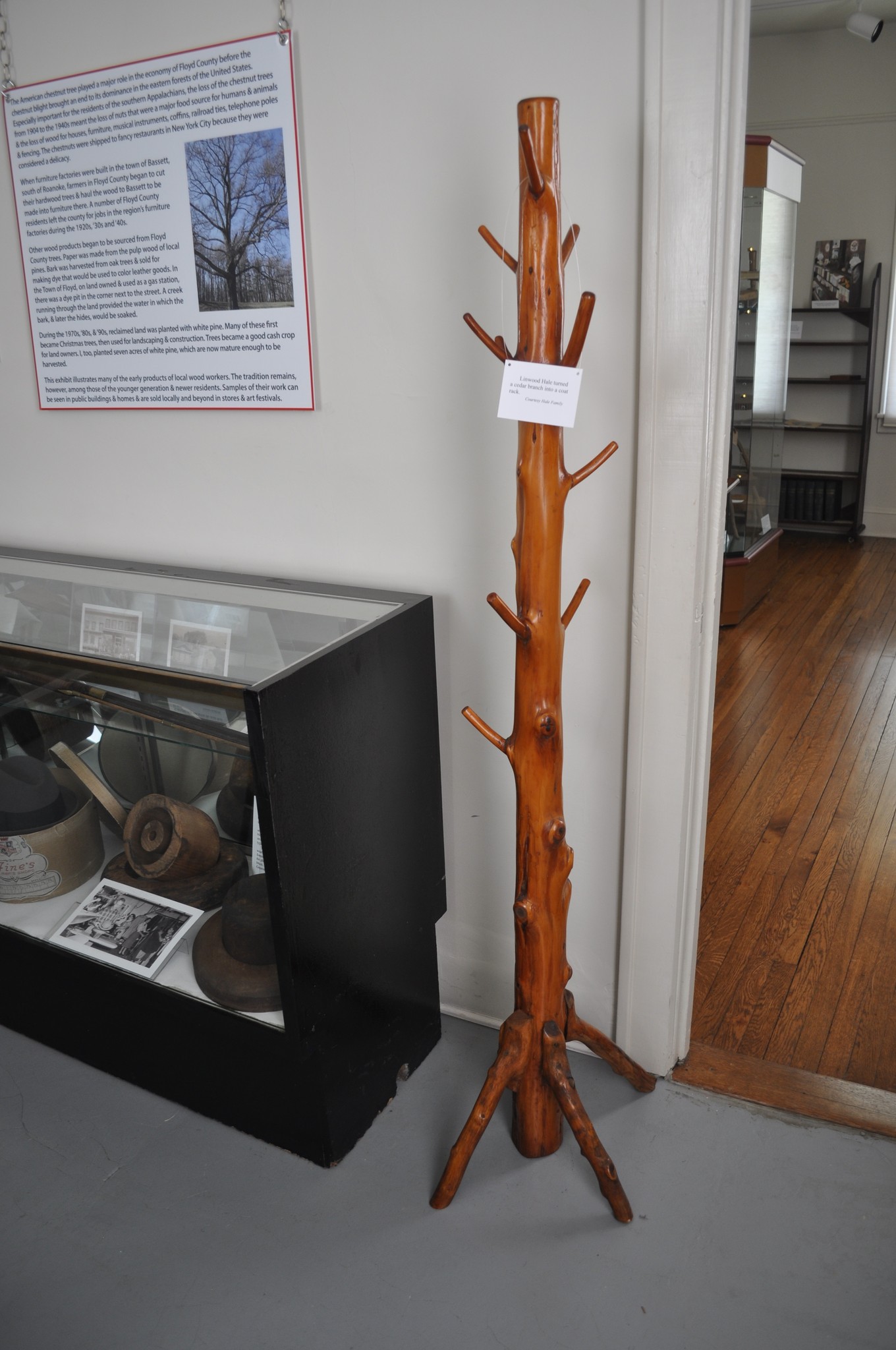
[[0, 547, 446, 1170], [726, 260, 882, 532]]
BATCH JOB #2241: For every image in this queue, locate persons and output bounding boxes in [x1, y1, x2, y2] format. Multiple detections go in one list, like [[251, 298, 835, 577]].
[[91, 897, 176, 967]]
